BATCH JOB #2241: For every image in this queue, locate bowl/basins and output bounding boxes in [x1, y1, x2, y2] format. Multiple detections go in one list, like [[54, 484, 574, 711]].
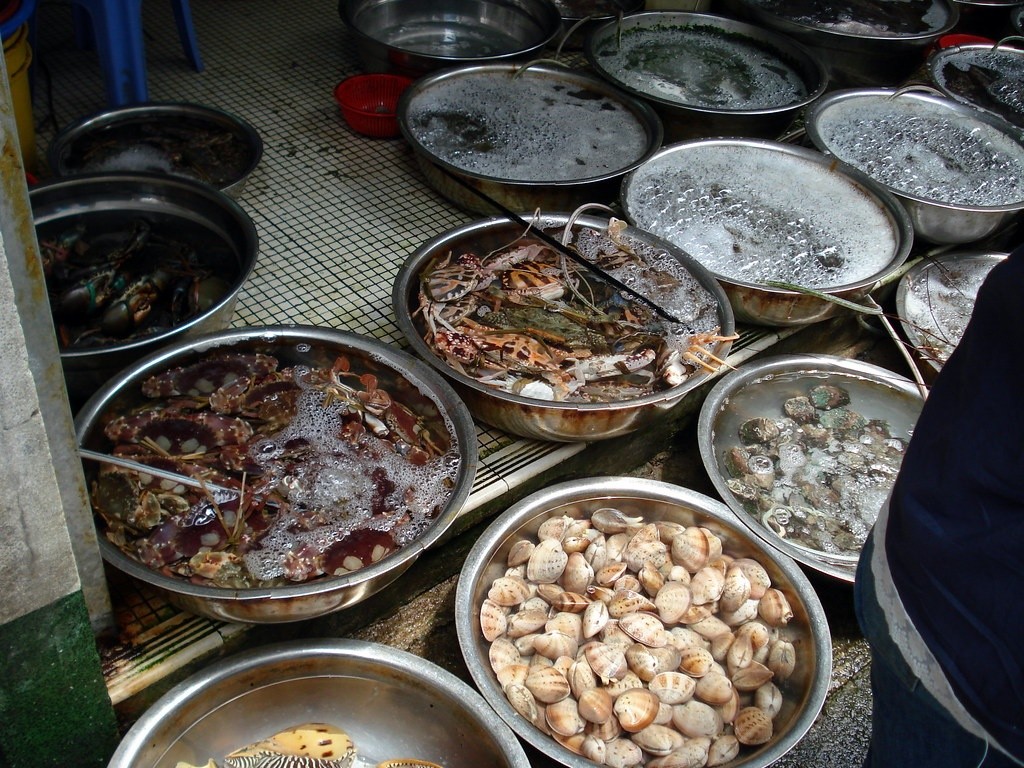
[[953, 0, 1024, 33], [896, 249, 1013, 374], [33, 100, 263, 203], [583, 10, 829, 147], [391, 208, 735, 442], [106, 636, 532, 768], [0, 0, 37, 44], [28, 169, 260, 416], [697, 352, 930, 585], [74, 323, 477, 624], [454, 477, 833, 768], [396, 61, 664, 216], [925, 44, 1024, 129], [714, 0, 961, 94], [337, 0, 564, 81], [804, 86, 1024, 246], [550, 0, 645, 33], [618, 137, 914, 328]]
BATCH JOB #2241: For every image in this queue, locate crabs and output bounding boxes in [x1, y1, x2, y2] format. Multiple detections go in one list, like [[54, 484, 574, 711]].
[[413, 217, 742, 404], [38, 212, 234, 349], [87, 339, 454, 588], [68, 113, 251, 190]]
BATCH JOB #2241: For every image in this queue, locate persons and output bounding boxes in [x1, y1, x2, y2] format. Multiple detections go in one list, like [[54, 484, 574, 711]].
[[851, 245, 1024, 768]]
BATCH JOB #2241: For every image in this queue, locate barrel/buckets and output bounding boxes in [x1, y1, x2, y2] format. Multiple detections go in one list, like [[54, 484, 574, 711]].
[[3, 21, 38, 176]]
